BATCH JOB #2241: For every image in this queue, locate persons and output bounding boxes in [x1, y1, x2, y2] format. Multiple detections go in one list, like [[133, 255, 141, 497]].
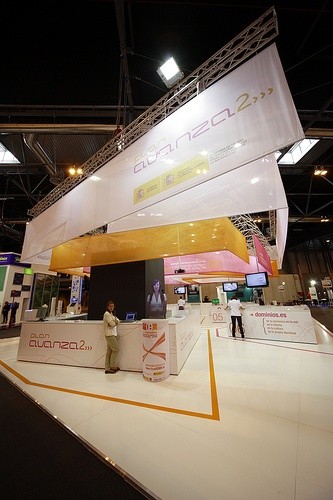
[[177, 296, 185, 310], [2, 301, 10, 323], [147, 279, 167, 320], [103, 299, 121, 375], [225, 295, 246, 341], [12, 302, 19, 323]]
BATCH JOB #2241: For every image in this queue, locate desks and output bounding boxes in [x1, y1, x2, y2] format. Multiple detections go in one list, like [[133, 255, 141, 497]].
[[18, 311, 201, 376]]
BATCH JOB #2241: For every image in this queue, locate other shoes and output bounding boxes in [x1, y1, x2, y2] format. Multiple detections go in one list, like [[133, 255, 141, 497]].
[[242, 337, 245, 341], [105, 367, 120, 374], [233, 336, 236, 340]]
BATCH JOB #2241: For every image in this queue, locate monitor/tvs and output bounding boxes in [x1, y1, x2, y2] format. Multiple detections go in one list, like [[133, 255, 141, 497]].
[[174, 287, 186, 294], [223, 282, 238, 292], [245, 271, 269, 288]]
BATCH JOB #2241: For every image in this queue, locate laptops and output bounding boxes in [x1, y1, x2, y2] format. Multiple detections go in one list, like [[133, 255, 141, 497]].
[[120, 312, 137, 323], [24, 309, 40, 323]]
